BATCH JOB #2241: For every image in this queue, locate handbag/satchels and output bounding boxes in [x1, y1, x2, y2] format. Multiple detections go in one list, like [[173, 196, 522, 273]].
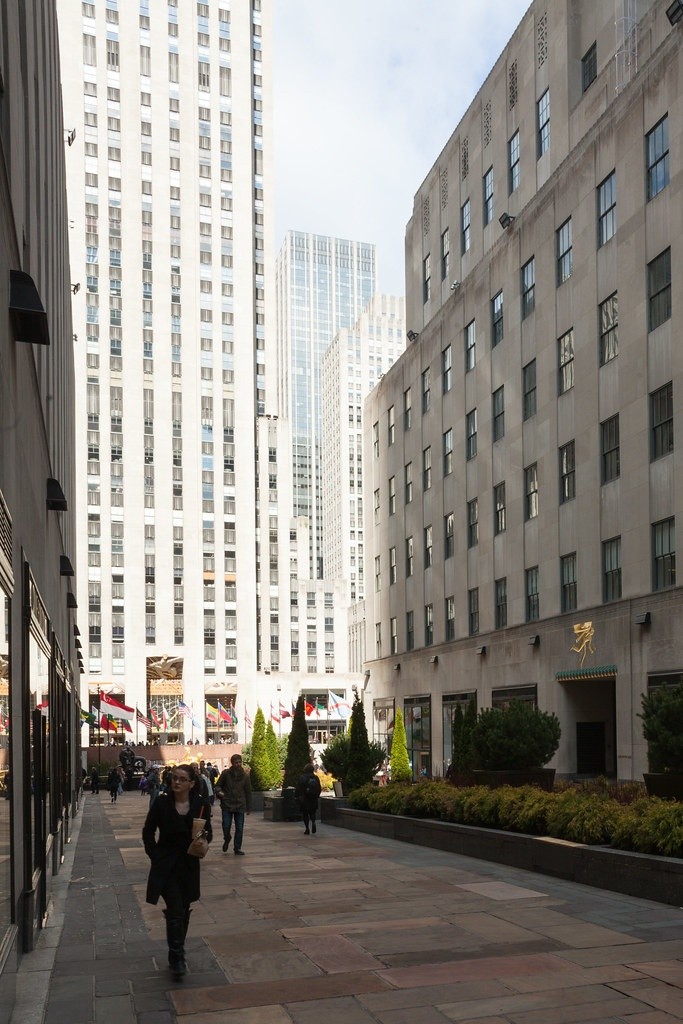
[[117, 783, 123, 795]]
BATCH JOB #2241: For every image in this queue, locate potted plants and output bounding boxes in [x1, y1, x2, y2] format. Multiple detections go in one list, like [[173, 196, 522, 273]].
[[636, 679, 683, 802], [469, 699, 562, 793], [248, 693, 413, 824]]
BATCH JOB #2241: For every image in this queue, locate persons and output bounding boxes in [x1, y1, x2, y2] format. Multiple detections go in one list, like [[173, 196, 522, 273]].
[[215, 753, 253, 855], [137, 760, 221, 818], [106, 766, 126, 804], [90, 767, 99, 794], [294, 764, 322, 834], [104, 735, 234, 744], [142, 764, 214, 974]]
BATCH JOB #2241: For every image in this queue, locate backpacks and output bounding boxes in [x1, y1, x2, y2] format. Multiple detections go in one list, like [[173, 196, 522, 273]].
[[302, 772, 317, 799]]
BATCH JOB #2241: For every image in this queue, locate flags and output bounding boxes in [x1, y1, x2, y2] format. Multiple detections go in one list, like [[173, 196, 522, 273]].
[[178, 701, 193, 719], [79, 688, 135, 736], [205, 702, 222, 726], [150, 708, 161, 730], [161, 706, 171, 731], [192, 715, 202, 729], [278, 701, 292, 718], [291, 703, 296, 721], [327, 689, 352, 712], [218, 702, 233, 724], [245, 707, 253, 729], [271, 704, 281, 722], [219, 703, 232, 724], [304, 701, 314, 716], [35, 700, 49, 717], [136, 709, 152, 729], [229, 704, 239, 724], [316, 703, 325, 717]]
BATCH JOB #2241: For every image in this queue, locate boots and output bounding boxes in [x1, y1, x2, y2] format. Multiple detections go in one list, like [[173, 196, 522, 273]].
[[163, 908, 185, 976], [183, 909, 194, 968]]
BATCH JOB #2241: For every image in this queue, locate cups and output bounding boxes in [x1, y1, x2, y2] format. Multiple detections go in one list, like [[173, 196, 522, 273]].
[[191, 817, 207, 839]]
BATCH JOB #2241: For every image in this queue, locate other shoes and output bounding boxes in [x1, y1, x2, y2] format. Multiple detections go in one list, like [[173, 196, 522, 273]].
[[112, 798, 114, 802], [304, 829, 310, 834], [223, 837, 231, 852], [311, 823, 317, 834], [234, 849, 244, 855]]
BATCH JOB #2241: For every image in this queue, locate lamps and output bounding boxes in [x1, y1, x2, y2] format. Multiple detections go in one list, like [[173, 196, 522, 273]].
[[429, 655, 439, 663], [75, 638, 82, 648], [364, 670, 370, 676], [60, 554, 75, 576], [79, 661, 84, 667], [77, 651, 83, 659], [393, 663, 400, 670], [66, 591, 77, 608], [527, 634, 540, 645], [80, 668, 84, 673], [7, 270, 51, 349], [46, 476, 68, 511], [476, 645, 487, 655], [633, 612, 651, 625], [73, 624, 80, 636]]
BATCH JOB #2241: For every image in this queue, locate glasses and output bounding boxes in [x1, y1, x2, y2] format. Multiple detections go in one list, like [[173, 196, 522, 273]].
[[171, 776, 191, 784]]
